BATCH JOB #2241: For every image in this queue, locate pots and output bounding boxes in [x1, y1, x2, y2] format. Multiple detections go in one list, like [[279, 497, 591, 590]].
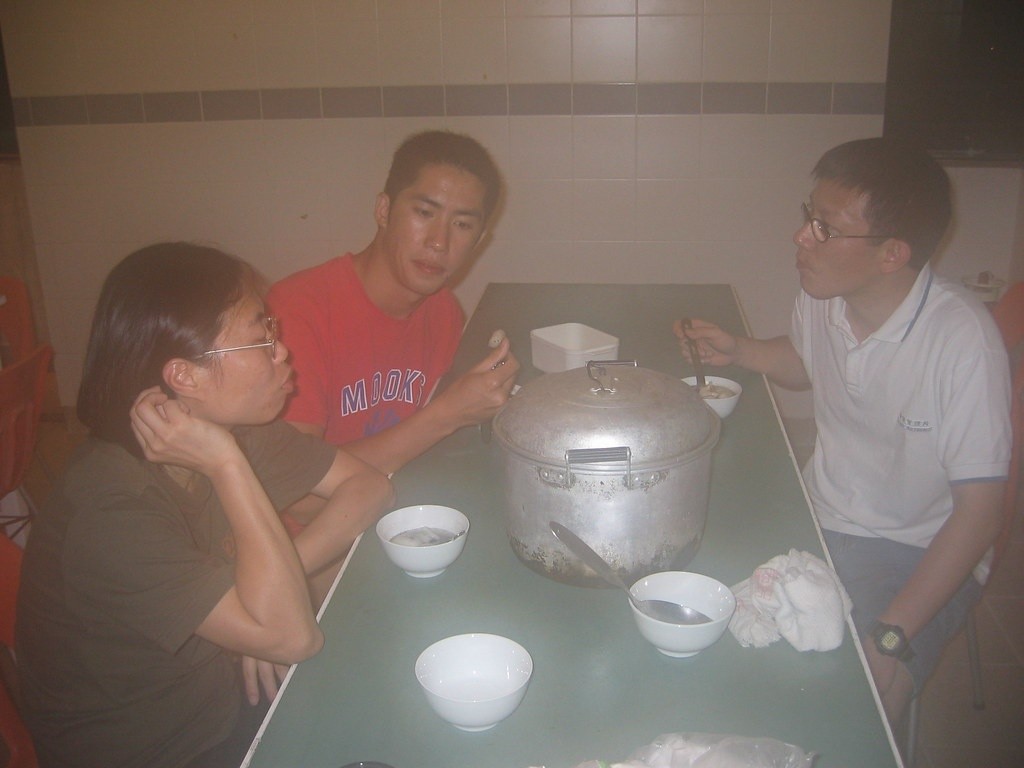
[[490, 360, 722, 589]]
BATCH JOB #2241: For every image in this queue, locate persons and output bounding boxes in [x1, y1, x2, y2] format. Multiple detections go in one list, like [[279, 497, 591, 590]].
[[271, 130, 520, 608], [16, 243, 394, 767], [673, 137, 1012, 727]]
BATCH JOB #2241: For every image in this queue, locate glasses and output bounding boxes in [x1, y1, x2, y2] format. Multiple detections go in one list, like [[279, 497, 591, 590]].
[[801, 201, 886, 243], [191, 303, 278, 361]]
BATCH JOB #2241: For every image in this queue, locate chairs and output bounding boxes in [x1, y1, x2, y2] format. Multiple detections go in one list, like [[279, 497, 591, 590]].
[[908, 280, 1024, 764]]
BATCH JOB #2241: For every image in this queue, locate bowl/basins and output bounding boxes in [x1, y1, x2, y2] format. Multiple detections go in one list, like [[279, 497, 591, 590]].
[[414, 633, 533, 732], [509, 383, 521, 395], [681, 375, 742, 419], [628, 571, 736, 658], [530, 322, 618, 373], [376, 504, 470, 580]]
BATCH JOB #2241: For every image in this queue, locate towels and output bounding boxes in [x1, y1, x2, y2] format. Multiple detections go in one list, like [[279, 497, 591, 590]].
[[725, 545, 856, 654]]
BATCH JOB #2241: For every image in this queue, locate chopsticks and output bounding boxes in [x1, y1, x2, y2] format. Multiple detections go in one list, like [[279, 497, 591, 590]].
[[491, 358, 505, 371]]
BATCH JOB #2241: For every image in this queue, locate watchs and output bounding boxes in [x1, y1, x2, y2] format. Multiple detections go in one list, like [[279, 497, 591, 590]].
[[866, 619, 915, 662]]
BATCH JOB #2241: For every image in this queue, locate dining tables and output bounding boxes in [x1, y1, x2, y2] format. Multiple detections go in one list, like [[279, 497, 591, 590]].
[[234, 279, 905, 768]]
